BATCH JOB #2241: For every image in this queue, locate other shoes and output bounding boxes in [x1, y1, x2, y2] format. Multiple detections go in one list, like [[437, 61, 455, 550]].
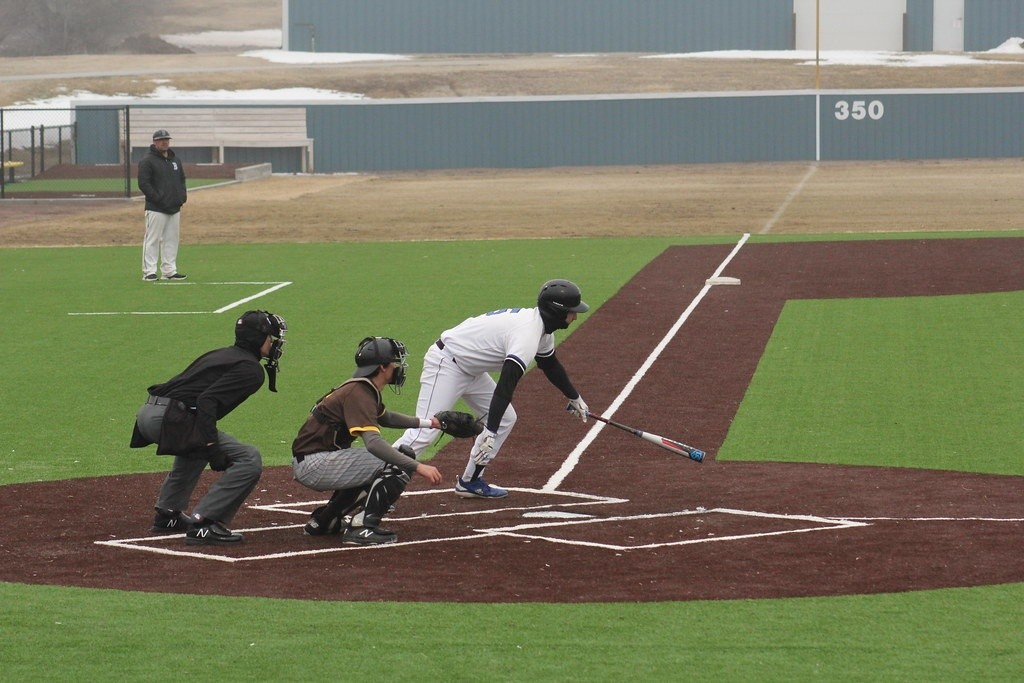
[[161, 273, 187, 280], [142, 274, 159, 282]]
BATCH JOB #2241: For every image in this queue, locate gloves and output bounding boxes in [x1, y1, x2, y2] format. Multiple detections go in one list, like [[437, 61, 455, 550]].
[[207, 443, 234, 472], [473, 429, 498, 466], [566, 393, 590, 423]]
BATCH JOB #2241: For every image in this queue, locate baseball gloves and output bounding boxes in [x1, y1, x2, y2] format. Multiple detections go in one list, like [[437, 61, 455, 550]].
[[433, 410, 485, 438]]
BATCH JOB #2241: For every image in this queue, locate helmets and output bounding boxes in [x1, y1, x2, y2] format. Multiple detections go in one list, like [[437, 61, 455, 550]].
[[234, 308, 286, 352], [537, 279, 590, 334], [351, 336, 405, 378]]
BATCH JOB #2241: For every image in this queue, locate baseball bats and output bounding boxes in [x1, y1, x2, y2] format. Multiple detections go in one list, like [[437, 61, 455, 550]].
[[566, 400, 706, 464]]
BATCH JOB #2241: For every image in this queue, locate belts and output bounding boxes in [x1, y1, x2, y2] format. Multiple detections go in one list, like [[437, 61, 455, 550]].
[[147, 395, 184, 411]]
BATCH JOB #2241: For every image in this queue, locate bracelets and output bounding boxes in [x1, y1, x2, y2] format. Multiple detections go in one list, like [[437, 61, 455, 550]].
[[419, 419, 432, 428]]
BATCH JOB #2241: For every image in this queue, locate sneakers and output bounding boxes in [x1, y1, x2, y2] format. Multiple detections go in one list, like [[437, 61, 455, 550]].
[[185, 516, 245, 546], [341, 523, 398, 546], [454, 474, 509, 499], [151, 508, 191, 535], [304, 511, 349, 537]]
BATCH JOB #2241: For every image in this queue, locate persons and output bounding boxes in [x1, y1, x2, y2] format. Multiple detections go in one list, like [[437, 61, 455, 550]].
[[292, 336, 485, 545], [387, 279, 588, 513], [138, 310, 285, 546], [138, 130, 186, 282]]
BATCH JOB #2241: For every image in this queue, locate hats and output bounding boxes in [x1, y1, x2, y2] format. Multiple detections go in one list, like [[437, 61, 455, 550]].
[[153, 129, 172, 141]]
